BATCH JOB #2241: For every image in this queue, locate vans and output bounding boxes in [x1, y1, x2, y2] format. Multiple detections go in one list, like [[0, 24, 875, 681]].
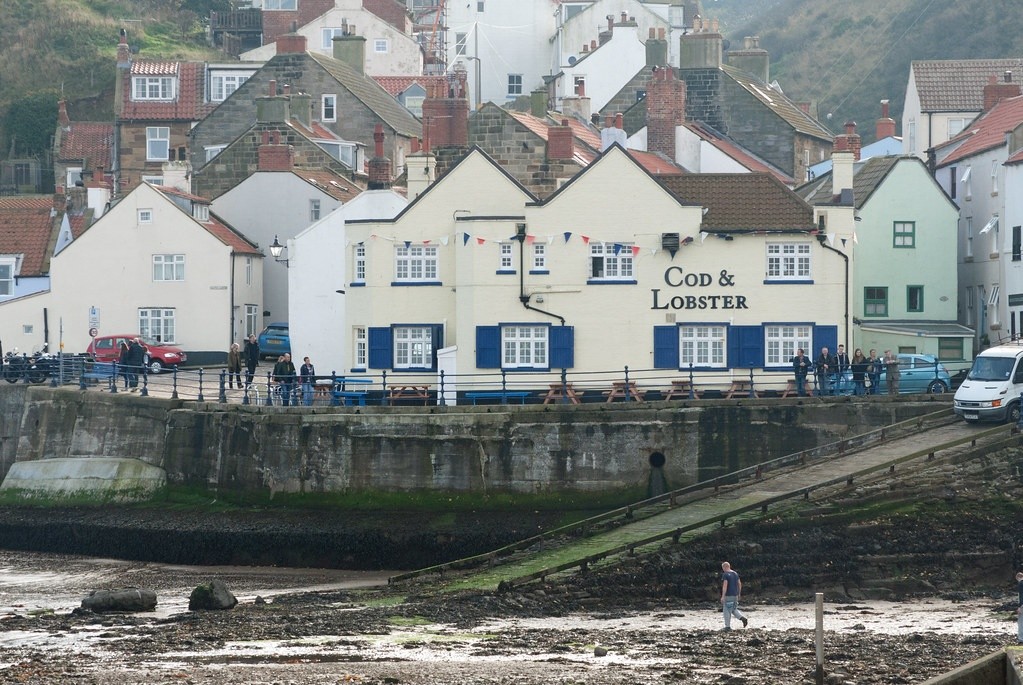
[[953, 333, 1023, 424]]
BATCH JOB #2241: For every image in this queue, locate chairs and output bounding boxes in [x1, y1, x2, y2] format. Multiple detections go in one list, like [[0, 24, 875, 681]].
[[242, 383, 302, 405]]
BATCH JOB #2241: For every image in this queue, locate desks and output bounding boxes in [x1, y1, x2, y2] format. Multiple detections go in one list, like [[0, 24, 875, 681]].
[[665, 380, 699, 400], [607, 381, 643, 402], [314, 383, 331, 399], [387, 384, 431, 406], [726, 380, 759, 399], [782, 379, 813, 397], [544, 384, 580, 404]]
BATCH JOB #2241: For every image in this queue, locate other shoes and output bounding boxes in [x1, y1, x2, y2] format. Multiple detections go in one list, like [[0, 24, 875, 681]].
[[131, 388, 137, 392], [742, 618, 747, 628], [238, 385, 243, 388]]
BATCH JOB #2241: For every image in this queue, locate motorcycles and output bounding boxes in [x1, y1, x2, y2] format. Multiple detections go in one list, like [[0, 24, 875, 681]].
[[0, 342, 59, 384]]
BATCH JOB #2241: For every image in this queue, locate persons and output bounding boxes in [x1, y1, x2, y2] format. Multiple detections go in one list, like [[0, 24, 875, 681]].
[[1015, 572, 1023, 646], [243, 334, 259, 389], [851, 349, 882, 396], [721, 562, 748, 630], [228, 343, 244, 390], [789, 352, 795, 362], [793, 349, 812, 397], [815, 344, 851, 396], [117, 336, 150, 394], [272, 352, 317, 406], [883, 350, 900, 394]]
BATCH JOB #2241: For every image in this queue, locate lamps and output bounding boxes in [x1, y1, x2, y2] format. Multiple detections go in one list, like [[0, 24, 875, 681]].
[[270, 234, 288, 268]]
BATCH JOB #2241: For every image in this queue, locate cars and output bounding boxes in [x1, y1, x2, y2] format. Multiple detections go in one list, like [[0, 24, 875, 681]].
[[825, 354, 951, 397], [258, 323, 291, 361], [86, 334, 188, 374]]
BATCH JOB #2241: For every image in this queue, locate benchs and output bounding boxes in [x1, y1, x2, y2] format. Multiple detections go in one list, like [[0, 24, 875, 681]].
[[466, 393, 532, 405], [330, 379, 373, 405], [539, 392, 584, 399], [780, 389, 814, 392], [602, 390, 648, 396], [721, 391, 757, 395], [386, 394, 430, 399], [661, 390, 705, 395], [80, 363, 121, 390]]
